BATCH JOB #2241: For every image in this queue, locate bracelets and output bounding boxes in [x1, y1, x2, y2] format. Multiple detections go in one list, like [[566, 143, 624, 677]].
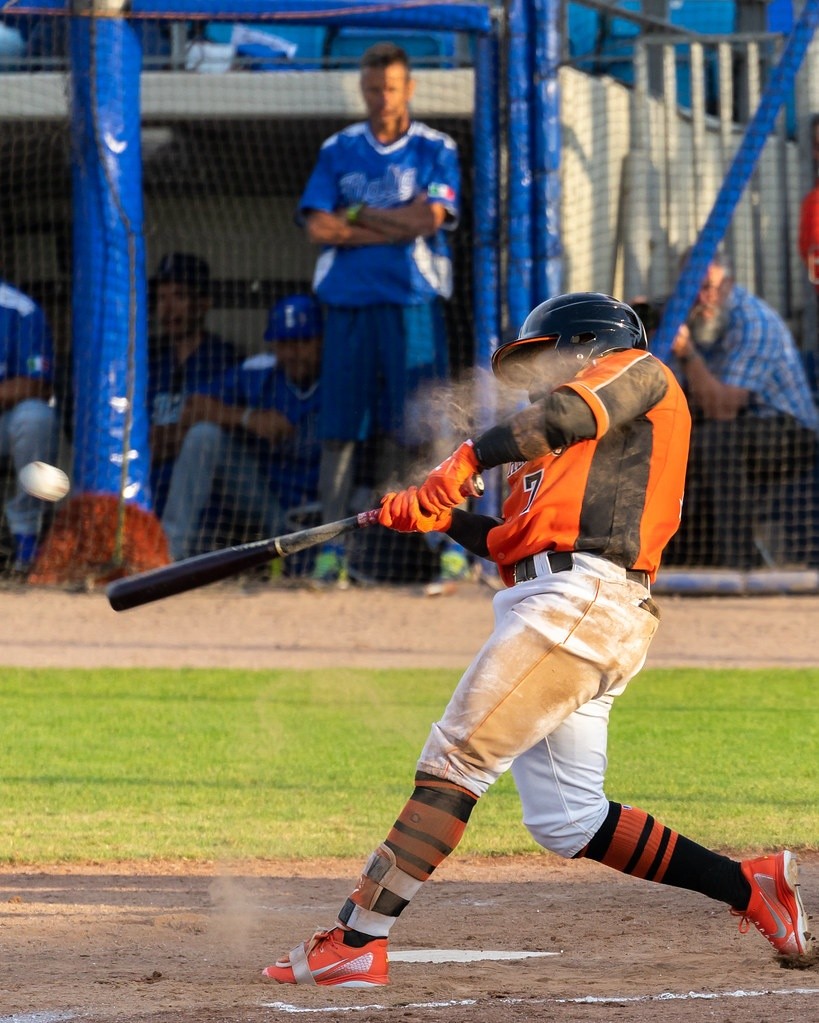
[[349, 200, 364, 224]]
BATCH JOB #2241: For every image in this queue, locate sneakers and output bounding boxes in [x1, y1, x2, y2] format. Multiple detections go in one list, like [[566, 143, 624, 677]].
[[308, 549, 350, 590], [424, 551, 471, 594], [261, 926, 390, 987], [729, 850, 808, 957]]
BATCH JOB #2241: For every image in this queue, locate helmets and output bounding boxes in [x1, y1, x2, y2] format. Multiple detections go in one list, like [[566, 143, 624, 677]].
[[491, 291, 648, 393]]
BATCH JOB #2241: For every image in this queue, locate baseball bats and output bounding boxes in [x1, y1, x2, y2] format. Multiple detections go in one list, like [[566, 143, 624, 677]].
[[103, 470, 486, 613]]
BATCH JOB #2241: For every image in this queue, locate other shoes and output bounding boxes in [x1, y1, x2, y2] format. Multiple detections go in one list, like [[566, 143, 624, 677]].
[[5, 560, 32, 590], [262, 556, 288, 584]]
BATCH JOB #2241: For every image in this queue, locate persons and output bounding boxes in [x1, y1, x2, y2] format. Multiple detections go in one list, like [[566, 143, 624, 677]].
[[161, 293, 374, 562], [673, 247, 818, 569], [295, 42, 475, 591], [145, 250, 248, 527], [0, 283, 61, 579], [262, 293, 814, 988]]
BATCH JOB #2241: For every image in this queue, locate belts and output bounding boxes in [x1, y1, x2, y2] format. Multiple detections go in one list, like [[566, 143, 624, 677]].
[[513, 550, 649, 589]]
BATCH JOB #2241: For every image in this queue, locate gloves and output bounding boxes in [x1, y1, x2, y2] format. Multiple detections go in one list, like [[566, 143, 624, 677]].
[[378, 485, 453, 533], [416, 439, 479, 515]]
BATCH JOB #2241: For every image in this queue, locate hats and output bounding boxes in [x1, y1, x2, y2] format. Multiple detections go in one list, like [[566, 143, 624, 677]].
[[262, 293, 321, 342]]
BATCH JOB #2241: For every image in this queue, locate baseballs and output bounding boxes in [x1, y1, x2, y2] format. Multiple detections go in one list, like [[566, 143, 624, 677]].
[[16, 460, 71, 504]]
